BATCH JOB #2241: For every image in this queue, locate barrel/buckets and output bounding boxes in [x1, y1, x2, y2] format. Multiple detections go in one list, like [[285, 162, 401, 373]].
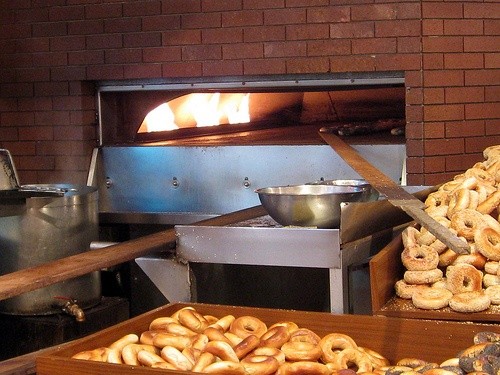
[[1, 184, 100, 318]]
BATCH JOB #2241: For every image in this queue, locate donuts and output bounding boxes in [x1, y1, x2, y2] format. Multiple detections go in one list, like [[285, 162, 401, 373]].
[[71, 307, 500, 375], [394, 145, 500, 314]]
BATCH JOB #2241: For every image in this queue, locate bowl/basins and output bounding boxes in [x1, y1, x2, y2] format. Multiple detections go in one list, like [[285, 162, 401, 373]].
[[255, 177, 380, 228]]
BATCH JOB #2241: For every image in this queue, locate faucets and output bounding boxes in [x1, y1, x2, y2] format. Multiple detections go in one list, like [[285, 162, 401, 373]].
[[51, 295, 86, 322]]
